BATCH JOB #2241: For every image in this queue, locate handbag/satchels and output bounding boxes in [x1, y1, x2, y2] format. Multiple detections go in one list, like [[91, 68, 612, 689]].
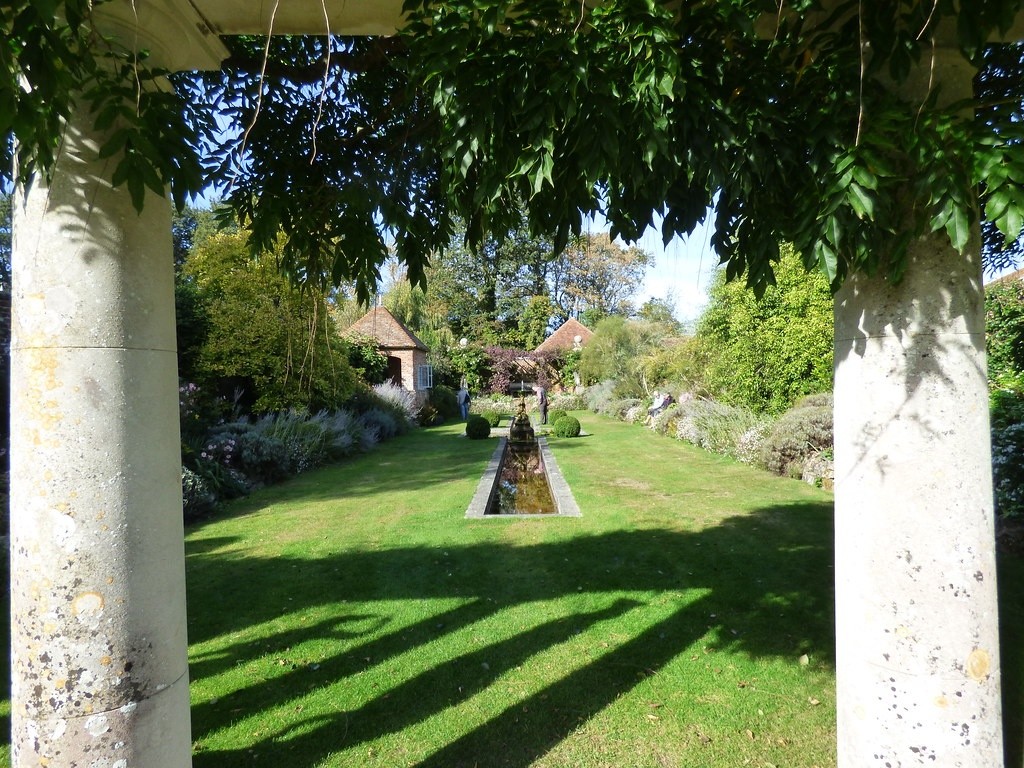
[[465, 393, 470, 403], [546, 397, 550, 406]]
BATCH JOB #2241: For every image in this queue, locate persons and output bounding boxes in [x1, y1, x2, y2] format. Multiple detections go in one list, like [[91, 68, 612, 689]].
[[457, 388, 470, 422], [538, 383, 548, 424]]
